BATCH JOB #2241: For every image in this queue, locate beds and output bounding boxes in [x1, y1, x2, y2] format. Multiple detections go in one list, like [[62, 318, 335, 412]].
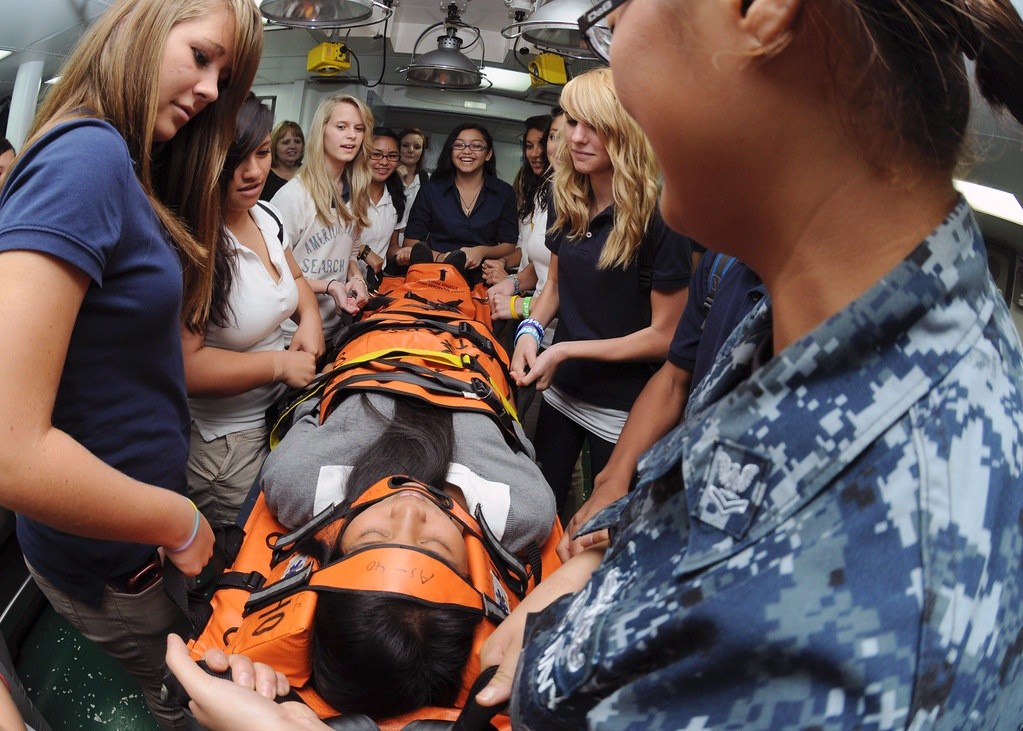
[[161, 258, 564, 731]]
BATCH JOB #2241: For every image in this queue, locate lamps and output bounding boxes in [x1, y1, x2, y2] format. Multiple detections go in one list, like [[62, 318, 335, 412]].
[[501, 0, 613, 61], [259, 0, 393, 29], [395, 0, 493, 92]]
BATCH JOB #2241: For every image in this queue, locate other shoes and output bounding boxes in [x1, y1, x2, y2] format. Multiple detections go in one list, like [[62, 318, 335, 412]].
[[443, 250, 467, 273], [409, 242, 434, 263]]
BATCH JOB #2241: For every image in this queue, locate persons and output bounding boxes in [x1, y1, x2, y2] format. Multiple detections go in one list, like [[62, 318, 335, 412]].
[[165, 0, 1023, 731], [260, 244, 557, 716], [259, 120, 305, 202], [269, 94, 375, 351], [486, 106, 593, 505], [403, 121, 519, 291], [556, 248, 762, 564], [397, 128, 432, 247], [358, 127, 408, 293], [507, 71, 705, 512], [177, 91, 327, 525], [0, 0, 263, 731], [481, 114, 553, 356]]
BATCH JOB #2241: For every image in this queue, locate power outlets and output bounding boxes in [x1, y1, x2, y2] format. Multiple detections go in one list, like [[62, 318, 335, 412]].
[[334, 48, 347, 61], [509, 0, 533, 20]]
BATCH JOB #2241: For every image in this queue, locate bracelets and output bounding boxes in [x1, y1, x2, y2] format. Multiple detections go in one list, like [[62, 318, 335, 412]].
[[508, 273, 519, 295], [326, 279, 336, 294], [358, 244, 371, 260], [351, 275, 368, 295], [435, 252, 442, 262], [511, 296, 546, 349], [164, 496, 200, 553]]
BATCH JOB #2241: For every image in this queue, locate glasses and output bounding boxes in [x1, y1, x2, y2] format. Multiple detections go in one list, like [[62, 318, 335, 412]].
[[368, 152, 401, 162], [451, 141, 488, 151], [577, 0, 625, 66]]
